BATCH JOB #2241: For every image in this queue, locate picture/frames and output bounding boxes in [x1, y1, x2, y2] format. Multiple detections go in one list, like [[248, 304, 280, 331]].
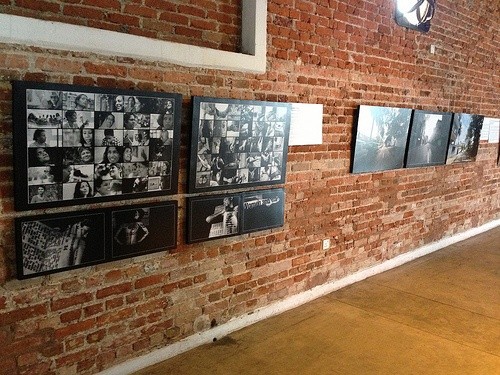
[[351, 104, 484, 174], [9, 76, 183, 212], [183, 187, 285, 245], [11, 198, 178, 280], [188, 93, 291, 196]]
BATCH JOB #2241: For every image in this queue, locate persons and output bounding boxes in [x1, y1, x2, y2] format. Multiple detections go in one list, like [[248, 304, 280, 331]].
[[27, 108, 63, 128], [123, 147, 132, 162], [132, 164, 138, 177], [78, 147, 93, 164], [115, 96, 123, 111], [165, 100, 173, 114], [135, 97, 149, 112], [113, 210, 149, 248], [156, 114, 174, 130], [46, 91, 62, 109], [94, 180, 112, 197], [104, 114, 117, 129], [123, 112, 135, 129], [101, 129, 122, 146], [101, 147, 122, 163], [139, 162, 148, 176], [30, 185, 51, 203], [160, 130, 172, 145], [127, 97, 136, 112], [196, 102, 288, 186], [109, 164, 122, 180], [63, 165, 72, 182], [28, 148, 56, 166], [162, 162, 170, 175], [71, 219, 92, 264], [72, 94, 88, 110], [205, 196, 239, 223], [134, 133, 145, 146], [143, 130, 149, 145], [63, 110, 88, 128], [71, 182, 93, 199]]
